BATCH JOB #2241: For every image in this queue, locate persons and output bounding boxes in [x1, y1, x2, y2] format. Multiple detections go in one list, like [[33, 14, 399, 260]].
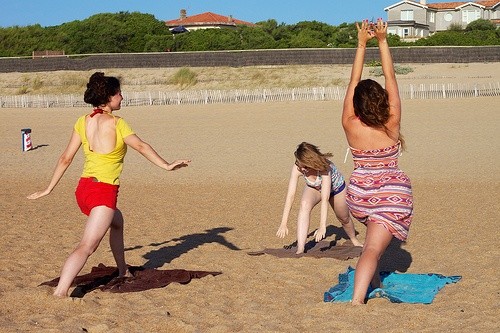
[[341, 19, 410, 307], [275, 142, 364, 255], [25, 72, 192, 297]]
